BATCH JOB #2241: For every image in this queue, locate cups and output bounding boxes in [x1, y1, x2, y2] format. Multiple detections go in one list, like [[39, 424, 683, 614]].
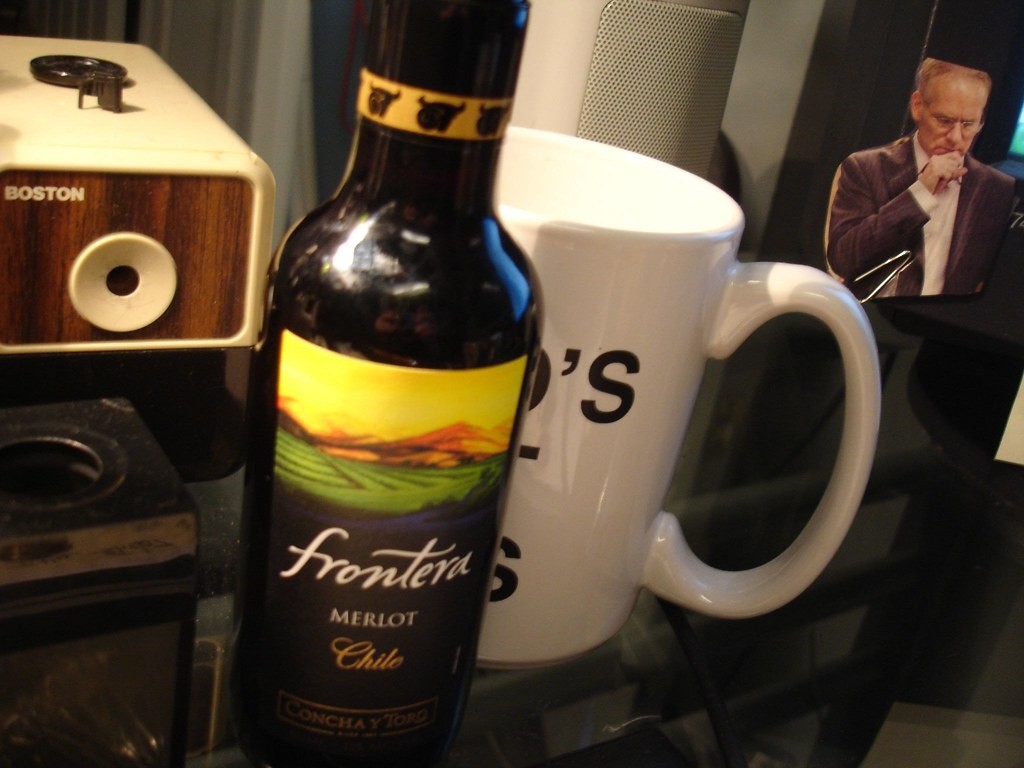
[[473, 127, 882, 662]]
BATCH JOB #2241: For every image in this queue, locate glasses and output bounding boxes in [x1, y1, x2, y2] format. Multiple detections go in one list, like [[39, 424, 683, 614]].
[[923, 104, 983, 133]]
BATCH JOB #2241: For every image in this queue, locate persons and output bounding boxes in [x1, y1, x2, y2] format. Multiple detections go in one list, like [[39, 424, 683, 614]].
[[826, 58, 1017, 299]]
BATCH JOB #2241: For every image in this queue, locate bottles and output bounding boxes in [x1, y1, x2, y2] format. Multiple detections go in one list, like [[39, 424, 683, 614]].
[[229, 0, 545, 768]]
